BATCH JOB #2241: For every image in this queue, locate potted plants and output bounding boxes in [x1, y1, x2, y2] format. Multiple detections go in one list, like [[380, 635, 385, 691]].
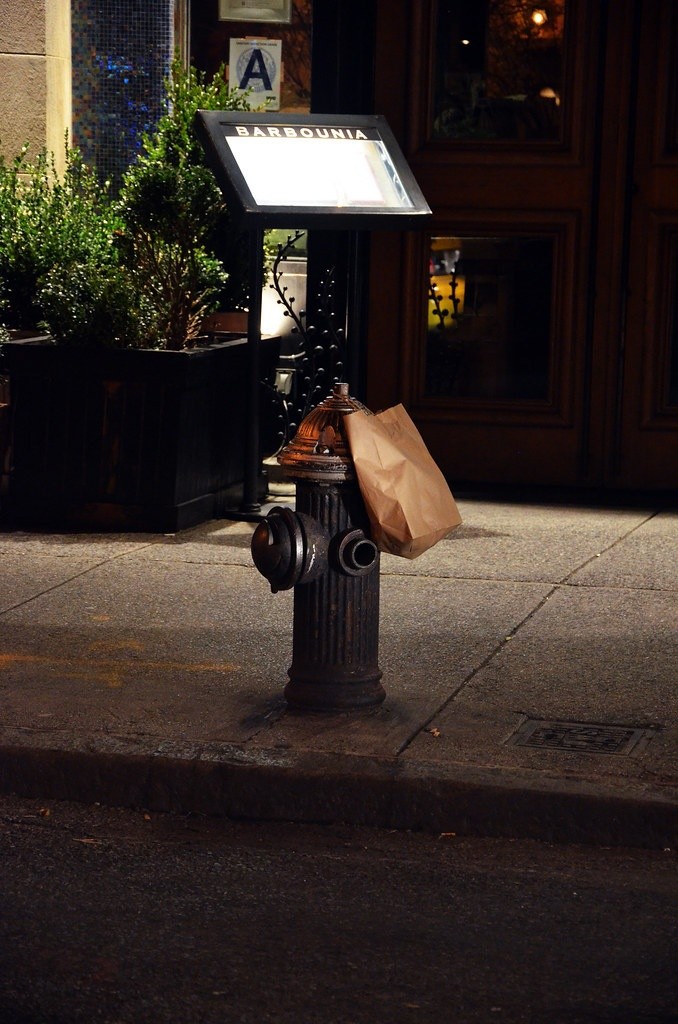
[[0, 132, 112, 526], [72, 47, 274, 532]]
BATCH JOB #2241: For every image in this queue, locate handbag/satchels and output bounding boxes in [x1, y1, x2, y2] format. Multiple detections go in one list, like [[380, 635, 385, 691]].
[[333, 390, 462, 559]]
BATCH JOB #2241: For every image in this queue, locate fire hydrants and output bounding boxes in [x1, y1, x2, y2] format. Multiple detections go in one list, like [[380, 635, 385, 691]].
[[250, 381, 388, 714]]
